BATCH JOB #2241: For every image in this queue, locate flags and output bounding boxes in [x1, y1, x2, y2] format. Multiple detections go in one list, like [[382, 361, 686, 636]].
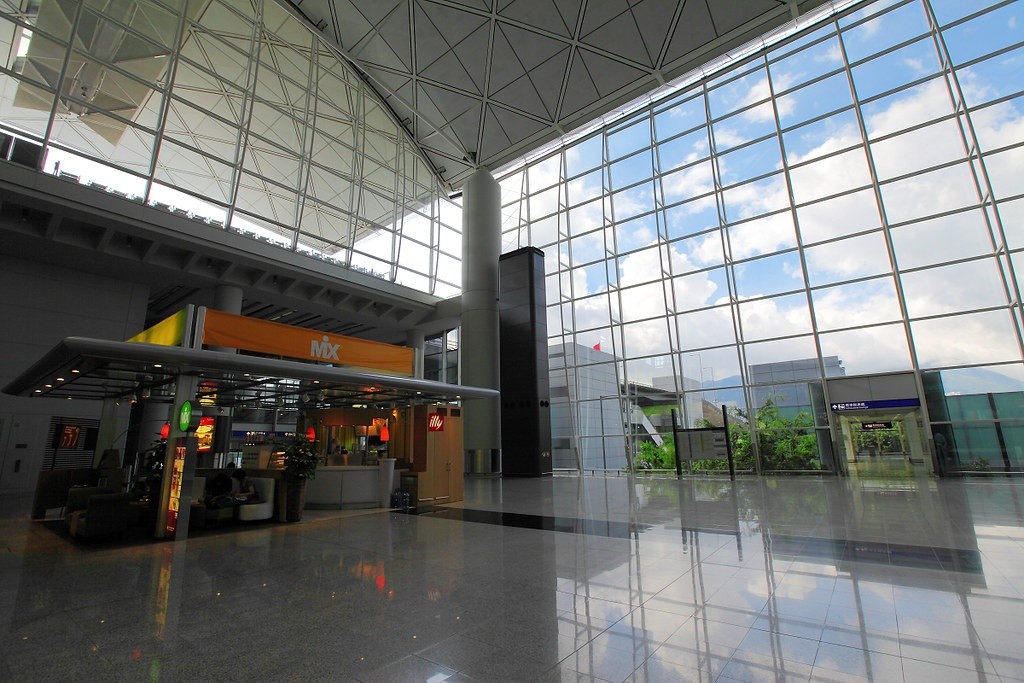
[[593, 343, 600, 351]]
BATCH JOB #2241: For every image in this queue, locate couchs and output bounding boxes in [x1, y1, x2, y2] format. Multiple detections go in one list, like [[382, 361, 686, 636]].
[[190, 477, 275, 521], [31, 472, 106, 538], [64, 486, 132, 544]]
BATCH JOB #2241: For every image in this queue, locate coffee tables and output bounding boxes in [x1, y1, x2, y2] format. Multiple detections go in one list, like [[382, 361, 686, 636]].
[[190, 503, 235, 531]]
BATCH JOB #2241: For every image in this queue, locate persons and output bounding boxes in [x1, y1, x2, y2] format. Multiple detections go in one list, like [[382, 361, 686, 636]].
[[340, 446, 349, 455], [229, 467, 260, 505], [226, 462, 235, 467], [332, 444, 341, 455], [204, 473, 232, 509]]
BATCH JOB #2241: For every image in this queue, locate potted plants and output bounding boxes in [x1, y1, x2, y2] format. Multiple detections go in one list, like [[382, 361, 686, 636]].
[[282, 435, 320, 523]]
